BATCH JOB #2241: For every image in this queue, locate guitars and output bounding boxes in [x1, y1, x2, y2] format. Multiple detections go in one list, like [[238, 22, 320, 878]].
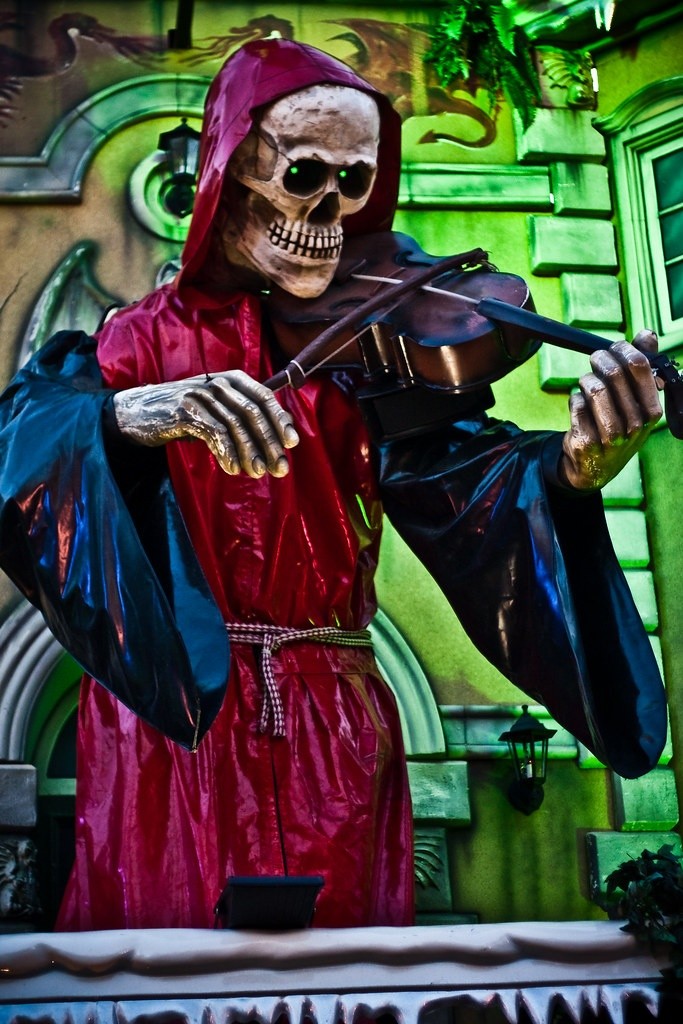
[[268, 230, 682, 443]]
